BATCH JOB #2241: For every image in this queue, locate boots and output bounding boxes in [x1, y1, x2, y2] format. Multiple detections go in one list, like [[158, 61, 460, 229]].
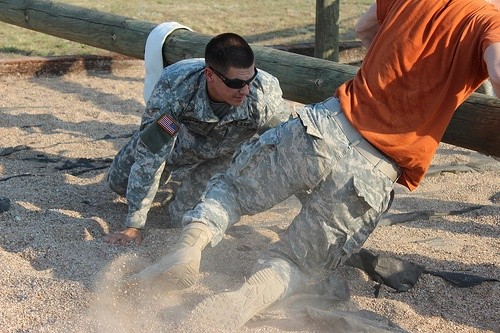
[[126, 224, 211, 288], [192, 268, 286, 333]]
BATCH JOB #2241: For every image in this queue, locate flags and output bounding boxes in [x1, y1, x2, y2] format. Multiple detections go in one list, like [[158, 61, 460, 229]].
[[158, 114, 180, 136]]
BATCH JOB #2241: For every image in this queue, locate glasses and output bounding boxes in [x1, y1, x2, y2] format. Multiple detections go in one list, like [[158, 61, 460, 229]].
[[209, 66, 258, 88]]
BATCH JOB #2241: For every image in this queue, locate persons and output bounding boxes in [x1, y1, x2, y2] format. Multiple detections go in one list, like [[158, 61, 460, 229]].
[[128, 0, 500, 333], [104, 31, 312, 247]]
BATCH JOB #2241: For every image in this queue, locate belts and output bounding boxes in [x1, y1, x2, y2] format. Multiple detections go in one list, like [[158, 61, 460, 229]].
[[324, 97, 402, 181]]
